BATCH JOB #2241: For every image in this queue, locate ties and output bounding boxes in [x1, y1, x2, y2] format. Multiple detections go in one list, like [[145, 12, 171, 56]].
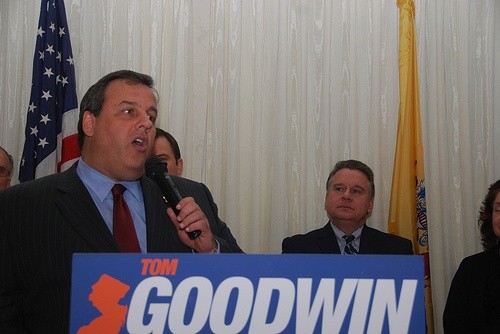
[[111, 184, 142, 253]]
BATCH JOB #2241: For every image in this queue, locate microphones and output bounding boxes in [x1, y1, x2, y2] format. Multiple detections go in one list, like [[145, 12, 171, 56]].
[[145, 157, 202, 240]]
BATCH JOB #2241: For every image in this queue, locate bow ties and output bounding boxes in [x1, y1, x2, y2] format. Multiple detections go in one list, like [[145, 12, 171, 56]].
[[342, 235, 357, 254]]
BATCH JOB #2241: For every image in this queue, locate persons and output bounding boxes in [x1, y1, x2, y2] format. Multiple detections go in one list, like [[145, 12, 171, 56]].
[[1, 70, 247, 334], [155, 126, 183, 178], [281, 158, 412, 256], [442, 179, 500, 334], [0, 144, 15, 190]]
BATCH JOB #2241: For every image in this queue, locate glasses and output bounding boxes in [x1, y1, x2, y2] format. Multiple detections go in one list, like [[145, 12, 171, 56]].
[[0, 166, 11, 177]]
